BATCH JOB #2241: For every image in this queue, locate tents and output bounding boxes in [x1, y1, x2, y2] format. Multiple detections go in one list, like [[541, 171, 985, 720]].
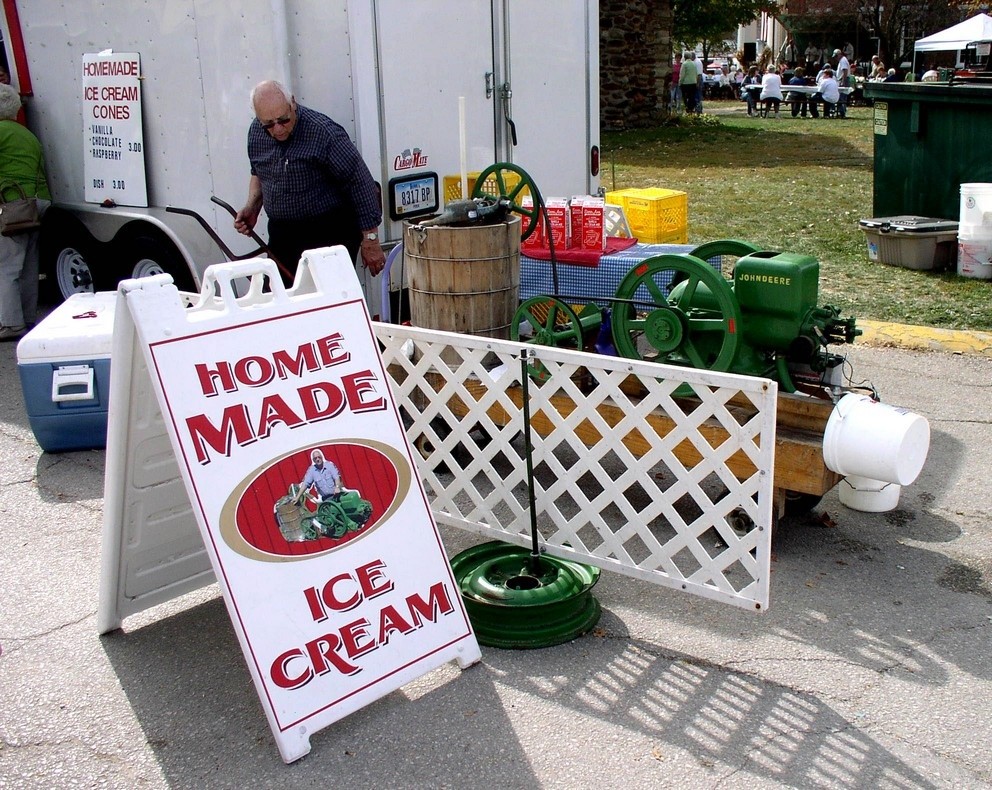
[[912, 12, 992, 84]]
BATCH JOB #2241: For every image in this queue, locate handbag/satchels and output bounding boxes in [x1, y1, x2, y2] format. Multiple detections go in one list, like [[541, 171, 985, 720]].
[[0, 178, 42, 234]]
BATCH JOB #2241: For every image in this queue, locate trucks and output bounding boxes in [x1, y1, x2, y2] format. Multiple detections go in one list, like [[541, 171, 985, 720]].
[[0, 0, 600, 303]]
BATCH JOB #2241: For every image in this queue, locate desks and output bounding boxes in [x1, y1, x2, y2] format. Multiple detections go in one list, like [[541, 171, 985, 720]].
[[745, 85, 855, 119], [520, 243, 722, 312]]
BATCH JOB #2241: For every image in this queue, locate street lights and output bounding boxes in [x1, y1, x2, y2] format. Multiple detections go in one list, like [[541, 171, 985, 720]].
[[870, 36, 880, 57]]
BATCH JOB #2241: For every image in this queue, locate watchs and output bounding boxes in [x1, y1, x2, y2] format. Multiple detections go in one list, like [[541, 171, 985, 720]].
[[363, 232, 379, 240]]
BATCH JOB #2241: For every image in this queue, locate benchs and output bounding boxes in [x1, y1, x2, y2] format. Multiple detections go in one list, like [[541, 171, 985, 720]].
[[753, 99, 852, 104]]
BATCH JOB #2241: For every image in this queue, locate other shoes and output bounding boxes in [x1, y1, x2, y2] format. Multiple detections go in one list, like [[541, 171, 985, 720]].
[[753, 111, 759, 116], [801, 116, 805, 118], [25, 323, 34, 329], [824, 116, 829, 119], [810, 115, 819, 118], [0, 326, 28, 341], [775, 113, 780, 119], [765, 113, 770, 118], [747, 114, 752, 118]]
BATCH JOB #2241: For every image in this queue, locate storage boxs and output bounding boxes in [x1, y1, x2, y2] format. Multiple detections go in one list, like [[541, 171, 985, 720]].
[[858, 216, 960, 270], [444, 172, 529, 216], [606, 187, 688, 246], [16, 290, 121, 455], [522, 196, 606, 251]]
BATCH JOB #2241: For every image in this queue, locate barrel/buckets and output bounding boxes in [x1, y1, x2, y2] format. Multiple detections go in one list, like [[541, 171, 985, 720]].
[[839, 476, 901, 512], [957, 183, 992, 278], [822, 393, 930, 486]]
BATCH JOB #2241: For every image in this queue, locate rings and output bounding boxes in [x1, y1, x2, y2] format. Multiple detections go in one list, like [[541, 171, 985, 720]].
[[381, 262, 385, 265]]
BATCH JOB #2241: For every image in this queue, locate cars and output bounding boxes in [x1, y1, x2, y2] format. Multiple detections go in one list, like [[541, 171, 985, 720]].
[[697, 56, 739, 74]]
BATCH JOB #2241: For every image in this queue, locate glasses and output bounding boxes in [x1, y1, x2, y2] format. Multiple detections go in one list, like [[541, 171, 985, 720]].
[[262, 103, 291, 129]]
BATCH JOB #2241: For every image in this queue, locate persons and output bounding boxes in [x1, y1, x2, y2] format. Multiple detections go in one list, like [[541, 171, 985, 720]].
[[235, 80, 387, 293], [294, 450, 340, 503], [0, 70, 53, 343], [671, 40, 940, 119]]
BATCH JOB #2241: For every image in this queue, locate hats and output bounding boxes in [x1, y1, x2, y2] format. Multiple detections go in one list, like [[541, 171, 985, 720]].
[[832, 48, 841, 57], [821, 63, 831, 69]]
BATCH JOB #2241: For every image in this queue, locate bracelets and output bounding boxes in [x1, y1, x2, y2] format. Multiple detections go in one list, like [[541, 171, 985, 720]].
[[336, 485, 340, 487]]
